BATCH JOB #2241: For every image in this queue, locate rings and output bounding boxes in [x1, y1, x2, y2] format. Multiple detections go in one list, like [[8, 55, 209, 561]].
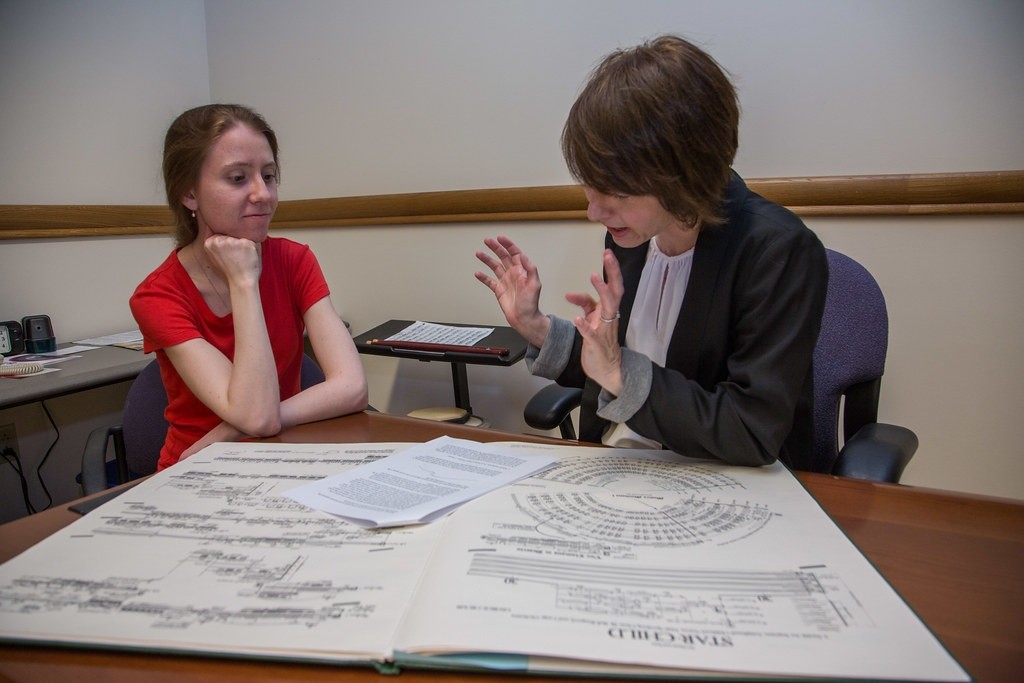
[[601, 311, 621, 324]]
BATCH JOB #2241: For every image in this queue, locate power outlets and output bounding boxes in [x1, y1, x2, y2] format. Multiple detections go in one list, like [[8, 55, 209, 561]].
[[0, 424, 21, 464]]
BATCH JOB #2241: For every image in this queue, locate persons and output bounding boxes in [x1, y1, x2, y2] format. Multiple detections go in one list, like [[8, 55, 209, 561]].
[[476, 33, 830, 471], [129, 104, 370, 472]]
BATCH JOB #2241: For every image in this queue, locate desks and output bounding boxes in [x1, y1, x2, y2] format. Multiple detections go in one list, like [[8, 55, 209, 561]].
[[0, 410, 1023, 682], [0, 321, 351, 410]]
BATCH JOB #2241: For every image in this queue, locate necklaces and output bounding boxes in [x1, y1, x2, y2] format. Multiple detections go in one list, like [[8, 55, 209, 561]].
[[191, 242, 232, 314]]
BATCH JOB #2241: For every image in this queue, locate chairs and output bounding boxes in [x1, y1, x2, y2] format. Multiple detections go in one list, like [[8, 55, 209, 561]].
[[524, 249, 918, 485], [79, 354, 380, 493]]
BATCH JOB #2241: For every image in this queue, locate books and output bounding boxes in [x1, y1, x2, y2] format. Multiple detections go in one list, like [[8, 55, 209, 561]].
[[0, 439, 975, 683]]
